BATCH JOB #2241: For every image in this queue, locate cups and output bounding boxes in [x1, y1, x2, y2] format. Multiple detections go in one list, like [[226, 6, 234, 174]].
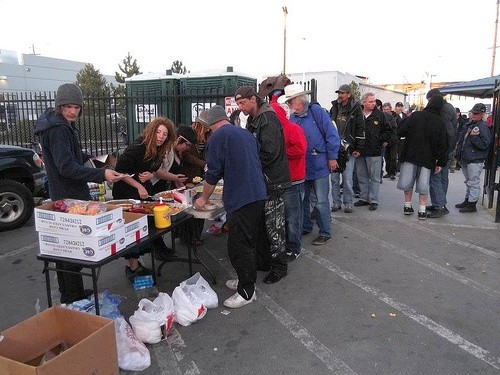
[[473, 127, 479, 133]]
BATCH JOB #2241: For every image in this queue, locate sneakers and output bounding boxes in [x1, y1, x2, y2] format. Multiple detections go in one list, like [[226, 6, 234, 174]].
[[257, 232, 332, 284]]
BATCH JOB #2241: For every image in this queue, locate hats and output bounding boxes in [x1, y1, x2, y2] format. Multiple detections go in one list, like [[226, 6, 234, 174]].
[[277, 84, 313, 104], [427, 88, 448, 98], [335, 84, 352, 93], [207, 105, 230, 125], [195, 110, 210, 128], [469, 103, 486, 114], [235, 86, 263, 101], [396, 102, 404, 106], [55, 84, 83, 108]]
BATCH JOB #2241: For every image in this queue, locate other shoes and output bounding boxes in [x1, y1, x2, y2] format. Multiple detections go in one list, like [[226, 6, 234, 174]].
[[60, 289, 96, 304], [226, 279, 257, 290], [125, 261, 161, 281], [155, 247, 179, 261], [223, 288, 256, 309], [331, 199, 477, 220], [383, 173, 397, 179]]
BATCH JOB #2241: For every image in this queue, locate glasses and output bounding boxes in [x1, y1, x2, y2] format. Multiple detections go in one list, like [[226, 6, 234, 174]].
[[185, 142, 193, 151]]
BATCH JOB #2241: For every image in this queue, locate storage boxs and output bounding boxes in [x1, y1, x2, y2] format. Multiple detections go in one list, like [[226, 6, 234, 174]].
[[0, 306, 121, 375], [35, 200, 124, 237], [124, 213, 149, 246], [39, 227, 126, 262]]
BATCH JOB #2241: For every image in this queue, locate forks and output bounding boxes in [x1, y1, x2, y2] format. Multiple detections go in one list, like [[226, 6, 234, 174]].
[[123, 174, 135, 177]]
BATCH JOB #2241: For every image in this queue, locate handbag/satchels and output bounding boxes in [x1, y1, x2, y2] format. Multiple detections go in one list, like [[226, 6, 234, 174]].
[[330, 143, 349, 174], [114, 317, 151, 371], [129, 272, 219, 344]]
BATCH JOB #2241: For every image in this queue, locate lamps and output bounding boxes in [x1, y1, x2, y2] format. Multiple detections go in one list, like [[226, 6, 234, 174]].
[[0, 76, 7, 81]]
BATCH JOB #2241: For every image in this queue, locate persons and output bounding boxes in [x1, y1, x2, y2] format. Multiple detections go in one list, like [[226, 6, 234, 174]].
[[111, 73, 500, 283], [353, 92, 387, 211], [195, 104, 268, 308], [32, 83, 130, 304]]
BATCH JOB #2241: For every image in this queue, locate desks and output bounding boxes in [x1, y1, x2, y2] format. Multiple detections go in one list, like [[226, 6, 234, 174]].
[[37, 213, 200, 317], [158, 177, 230, 284]]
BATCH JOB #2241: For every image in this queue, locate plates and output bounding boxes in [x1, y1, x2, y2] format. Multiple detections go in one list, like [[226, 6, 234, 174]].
[[105, 178, 224, 218]]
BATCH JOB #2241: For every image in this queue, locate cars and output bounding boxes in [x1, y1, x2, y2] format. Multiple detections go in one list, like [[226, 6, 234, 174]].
[[0, 144, 48, 230]]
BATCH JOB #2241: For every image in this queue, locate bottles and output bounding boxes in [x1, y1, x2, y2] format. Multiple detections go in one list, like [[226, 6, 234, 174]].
[[154, 202, 172, 228], [88, 182, 105, 203]]
